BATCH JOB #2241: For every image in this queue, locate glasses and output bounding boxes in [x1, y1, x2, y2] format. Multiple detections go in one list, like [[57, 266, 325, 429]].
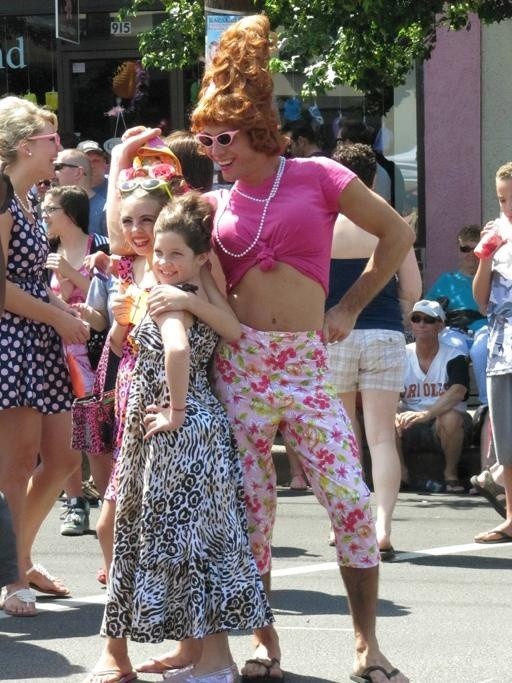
[[195, 130, 240, 147], [409, 314, 435, 325], [54, 163, 86, 176], [37, 179, 53, 187], [460, 245, 473, 253], [118, 178, 172, 200], [40, 206, 64, 214], [28, 132, 60, 145]]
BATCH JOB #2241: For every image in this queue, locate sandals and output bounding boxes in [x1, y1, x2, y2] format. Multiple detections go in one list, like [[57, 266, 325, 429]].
[[1, 565, 70, 617], [471, 467, 506, 519]]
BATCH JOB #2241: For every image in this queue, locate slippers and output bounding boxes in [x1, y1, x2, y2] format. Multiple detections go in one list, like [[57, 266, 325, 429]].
[[474, 529, 511, 544], [443, 472, 466, 493], [377, 544, 396, 563], [289, 474, 308, 492], [86, 655, 284, 683], [350, 667, 409, 683]]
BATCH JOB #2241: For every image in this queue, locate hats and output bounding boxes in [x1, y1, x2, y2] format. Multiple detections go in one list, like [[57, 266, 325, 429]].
[[77, 140, 106, 158], [412, 300, 446, 320]]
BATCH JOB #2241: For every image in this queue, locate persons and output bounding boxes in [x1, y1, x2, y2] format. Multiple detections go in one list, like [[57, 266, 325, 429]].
[[424, 225, 498, 496], [31, 122, 424, 584], [85, 188, 277, 683], [395, 299, 470, 494], [471, 160, 512, 543], [0, 94, 93, 617], [190, 14, 417, 681]]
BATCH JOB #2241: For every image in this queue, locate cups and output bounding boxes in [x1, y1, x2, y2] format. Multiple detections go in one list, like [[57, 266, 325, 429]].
[[472, 215, 512, 262]]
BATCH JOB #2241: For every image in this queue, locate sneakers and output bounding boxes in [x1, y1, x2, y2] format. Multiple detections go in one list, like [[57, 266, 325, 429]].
[[60, 479, 101, 536]]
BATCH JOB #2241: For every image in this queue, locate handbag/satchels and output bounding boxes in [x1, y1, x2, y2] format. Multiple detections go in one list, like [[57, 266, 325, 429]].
[[71, 390, 117, 456]]
